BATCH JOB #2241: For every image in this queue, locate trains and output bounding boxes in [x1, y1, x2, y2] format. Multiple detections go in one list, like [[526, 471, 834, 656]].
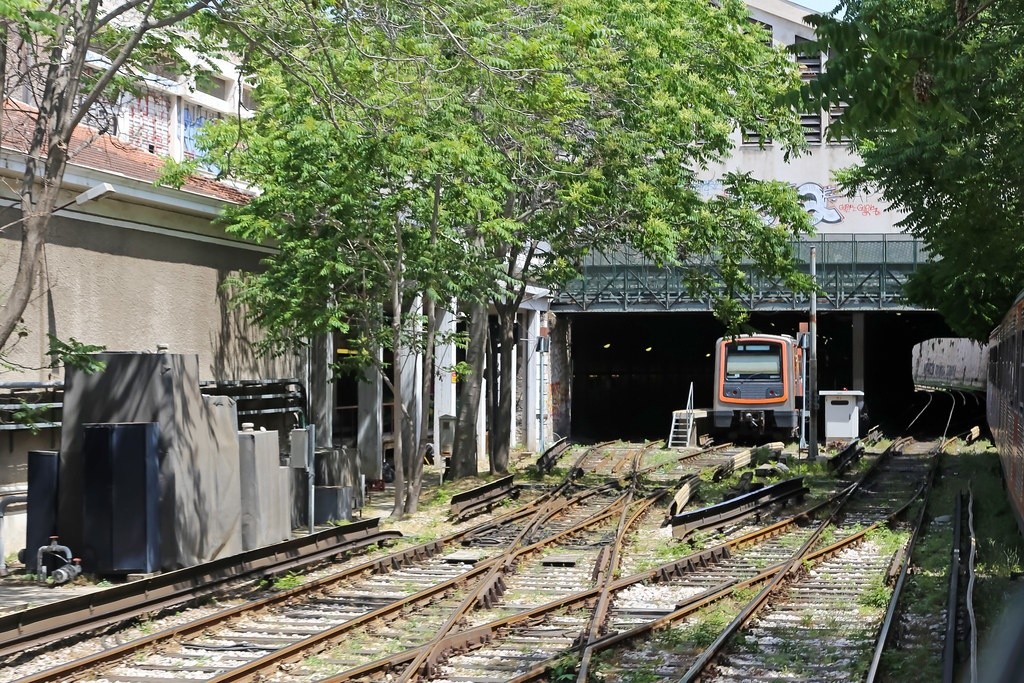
[[713, 332, 809, 441]]
